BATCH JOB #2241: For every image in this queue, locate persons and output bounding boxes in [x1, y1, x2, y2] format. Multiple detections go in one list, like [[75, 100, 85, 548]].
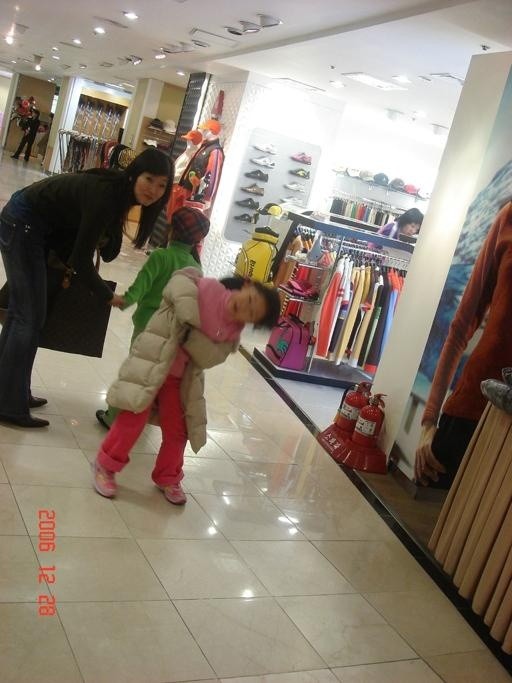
[[179, 119, 224, 256], [0, 147, 175, 427], [368, 208, 424, 250], [37, 112, 54, 166], [174, 130, 203, 184], [95, 206, 211, 429], [89, 266, 281, 506], [413, 199, 512, 487], [10, 109, 41, 162]]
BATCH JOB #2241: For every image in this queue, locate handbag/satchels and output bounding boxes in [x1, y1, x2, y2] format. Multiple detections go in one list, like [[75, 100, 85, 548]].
[[35, 237, 116, 359], [263, 313, 310, 370]]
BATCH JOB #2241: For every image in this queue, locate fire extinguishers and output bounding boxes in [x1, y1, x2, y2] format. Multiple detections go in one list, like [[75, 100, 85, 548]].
[[351, 393, 388, 447], [333, 381, 373, 432]]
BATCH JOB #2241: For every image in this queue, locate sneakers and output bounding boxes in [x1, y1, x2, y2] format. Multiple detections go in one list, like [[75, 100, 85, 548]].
[[155, 483, 187, 505], [288, 168, 311, 179], [290, 152, 312, 164], [234, 214, 255, 222], [10, 155, 18, 159], [25, 156, 29, 160], [280, 197, 302, 205], [92, 456, 117, 498], [255, 144, 279, 154], [284, 181, 306, 193], [96, 409, 112, 430], [244, 170, 268, 182], [250, 155, 276, 168]]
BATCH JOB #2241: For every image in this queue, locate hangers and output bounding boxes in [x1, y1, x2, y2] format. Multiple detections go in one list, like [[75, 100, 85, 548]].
[[292, 224, 318, 243], [338, 244, 409, 280]]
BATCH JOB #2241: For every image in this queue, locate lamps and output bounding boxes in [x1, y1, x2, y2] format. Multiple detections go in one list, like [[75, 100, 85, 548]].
[[132, 56, 143, 65], [181, 43, 193, 54], [172, 46, 181, 55], [194, 37, 207, 49], [155, 49, 169, 61], [34, 56, 42, 71], [242, 21, 260, 35], [259, 16, 281, 30], [230, 27, 242, 35]]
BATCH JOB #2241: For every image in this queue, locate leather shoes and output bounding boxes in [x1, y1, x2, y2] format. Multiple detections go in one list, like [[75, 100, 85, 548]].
[[241, 183, 264, 195], [28, 395, 47, 408], [235, 198, 259, 209], [1, 413, 50, 427]]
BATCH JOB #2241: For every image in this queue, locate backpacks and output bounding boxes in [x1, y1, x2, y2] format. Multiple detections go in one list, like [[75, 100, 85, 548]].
[[234, 226, 281, 290], [234, 240, 278, 289]]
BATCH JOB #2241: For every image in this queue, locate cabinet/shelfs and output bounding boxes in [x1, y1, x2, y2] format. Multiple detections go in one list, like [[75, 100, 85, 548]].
[[330, 212, 416, 244]]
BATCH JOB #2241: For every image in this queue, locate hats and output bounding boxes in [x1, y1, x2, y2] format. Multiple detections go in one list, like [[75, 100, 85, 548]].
[[144, 139, 157, 147], [148, 125, 162, 132], [332, 164, 346, 172], [158, 143, 169, 149], [361, 171, 374, 182], [288, 279, 312, 290], [418, 188, 424, 198], [404, 184, 417, 194], [151, 118, 162, 127], [389, 178, 404, 190], [181, 130, 202, 145], [163, 119, 177, 132], [166, 131, 176, 135], [173, 207, 210, 243], [33, 108, 40, 115], [347, 166, 362, 177], [260, 202, 283, 216], [374, 173, 388, 187], [198, 119, 221, 135]]
